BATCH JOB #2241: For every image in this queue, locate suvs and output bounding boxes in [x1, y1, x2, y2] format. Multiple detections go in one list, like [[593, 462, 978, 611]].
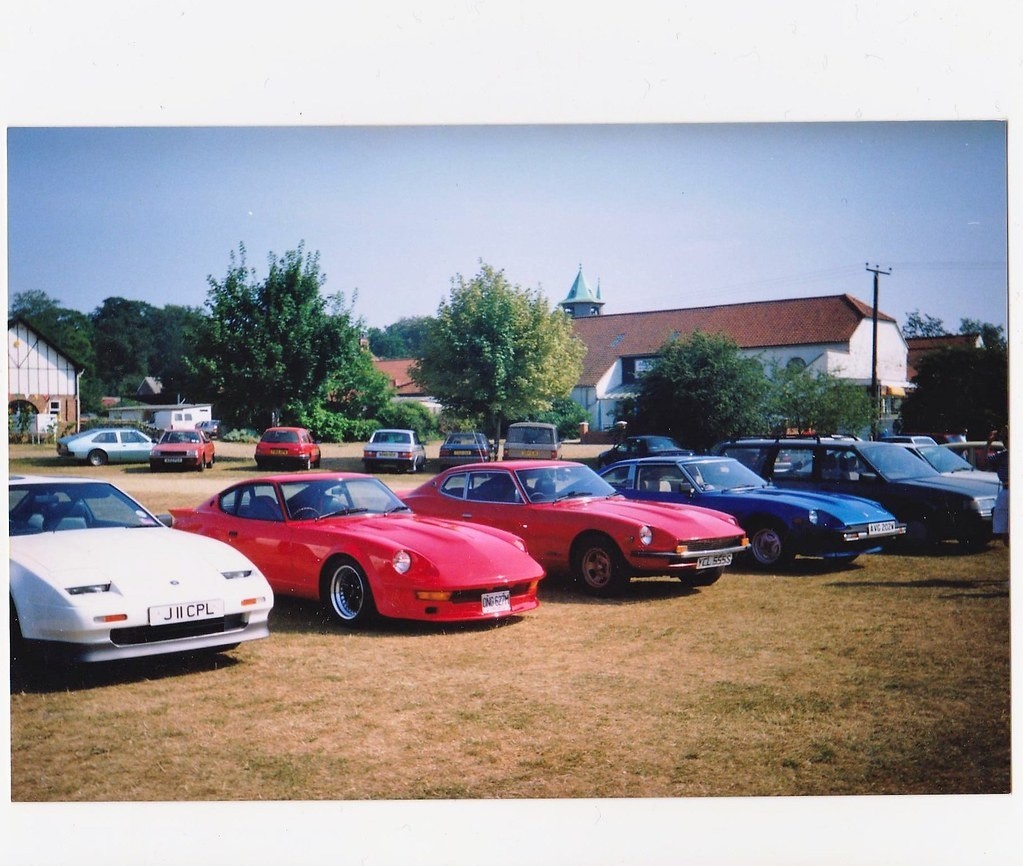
[[714, 433, 1006, 554]]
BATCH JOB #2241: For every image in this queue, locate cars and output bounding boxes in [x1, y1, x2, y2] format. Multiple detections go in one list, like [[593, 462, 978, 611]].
[[150, 428, 215, 472], [835, 430, 1009, 479], [359, 429, 428, 474], [438, 432, 495, 473], [597, 435, 696, 471], [392, 459, 751, 598], [57, 427, 156, 466], [194, 421, 222, 438], [254, 427, 321, 473], [167, 473, 546, 629], [8, 471, 275, 690], [557, 456, 908, 574]]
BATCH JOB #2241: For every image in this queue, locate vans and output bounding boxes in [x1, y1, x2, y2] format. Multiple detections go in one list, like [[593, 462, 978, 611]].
[[502, 422, 562, 462]]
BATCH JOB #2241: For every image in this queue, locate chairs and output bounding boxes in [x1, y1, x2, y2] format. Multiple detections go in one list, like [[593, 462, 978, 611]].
[[535, 478, 558, 502], [42, 501, 93, 532], [249, 495, 277, 521]]
